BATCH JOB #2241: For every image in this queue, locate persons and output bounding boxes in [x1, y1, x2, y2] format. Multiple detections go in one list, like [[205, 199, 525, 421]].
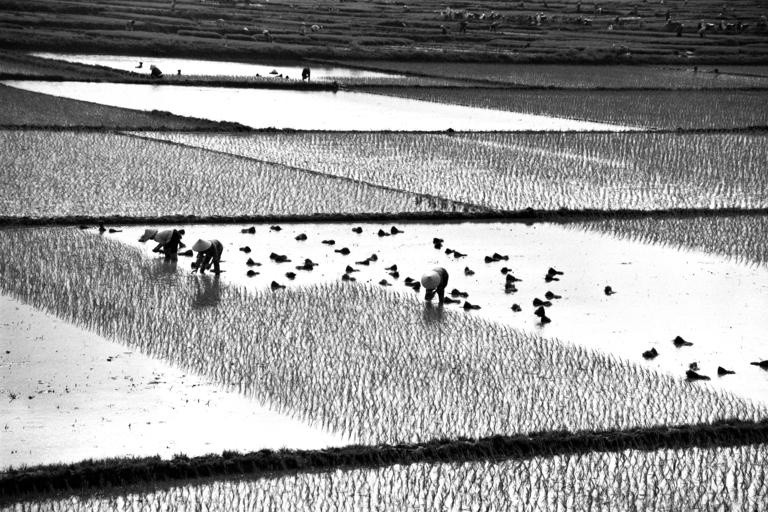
[[192, 238, 223, 276], [144, 229, 180, 263], [302, 67, 310, 81], [421, 266, 449, 305], [149, 64, 163, 78]]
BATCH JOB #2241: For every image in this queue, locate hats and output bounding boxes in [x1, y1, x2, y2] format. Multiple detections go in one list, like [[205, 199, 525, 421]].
[[150, 64, 155, 69], [192, 239, 212, 252], [421, 271, 441, 289], [145, 229, 158, 240]]
